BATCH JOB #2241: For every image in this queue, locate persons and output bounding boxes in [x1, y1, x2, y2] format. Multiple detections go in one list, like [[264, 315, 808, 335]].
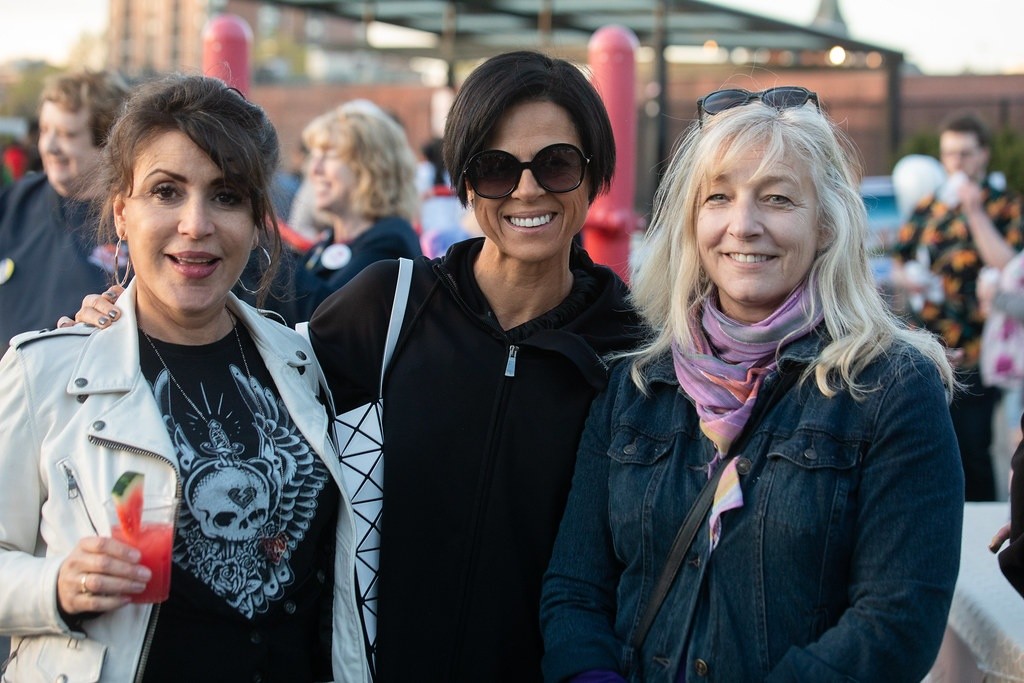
[[893, 119, 1023, 503], [60, 50, 657, 683], [1, 76, 457, 683], [541, 88, 965, 683]]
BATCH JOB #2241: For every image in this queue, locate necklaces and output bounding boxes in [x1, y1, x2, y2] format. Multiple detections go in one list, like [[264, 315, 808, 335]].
[[132, 307, 289, 569]]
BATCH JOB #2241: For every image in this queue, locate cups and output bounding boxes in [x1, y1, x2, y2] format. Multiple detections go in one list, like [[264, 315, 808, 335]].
[[104, 496, 179, 604]]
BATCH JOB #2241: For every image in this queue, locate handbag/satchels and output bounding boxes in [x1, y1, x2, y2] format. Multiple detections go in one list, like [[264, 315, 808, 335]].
[[1, 634, 107, 683], [294, 257, 414, 683]]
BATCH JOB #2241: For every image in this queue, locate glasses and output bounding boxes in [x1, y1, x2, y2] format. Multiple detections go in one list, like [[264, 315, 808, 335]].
[[697, 85, 820, 129], [464, 144, 593, 198]]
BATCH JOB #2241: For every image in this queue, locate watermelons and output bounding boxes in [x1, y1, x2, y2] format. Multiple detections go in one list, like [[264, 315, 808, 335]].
[[110, 471, 145, 538]]
